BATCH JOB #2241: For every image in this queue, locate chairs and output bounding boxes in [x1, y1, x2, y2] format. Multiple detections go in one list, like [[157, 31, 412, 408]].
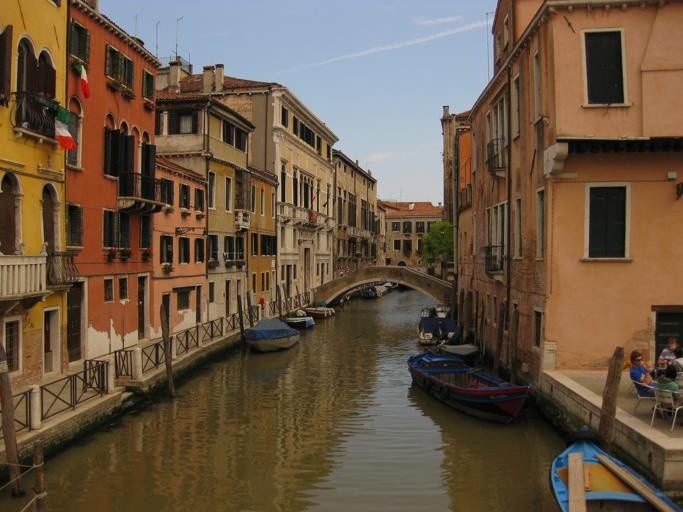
[[650, 388, 683, 433], [632, 379, 657, 416], [658, 359, 672, 370]]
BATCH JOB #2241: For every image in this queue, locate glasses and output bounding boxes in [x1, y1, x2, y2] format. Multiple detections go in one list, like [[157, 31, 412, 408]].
[[634, 358, 643, 362]]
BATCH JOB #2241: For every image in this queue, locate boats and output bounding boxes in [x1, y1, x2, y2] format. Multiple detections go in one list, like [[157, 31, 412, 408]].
[[363, 286, 389, 298], [286, 317, 314, 328], [245, 320, 301, 353], [417, 307, 479, 358], [408, 352, 534, 424], [305, 305, 336, 318], [550, 426, 681, 512]]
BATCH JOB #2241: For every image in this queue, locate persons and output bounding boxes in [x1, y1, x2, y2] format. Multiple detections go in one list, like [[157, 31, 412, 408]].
[[628, 348, 659, 397], [656, 336, 679, 369], [655, 363, 682, 427]]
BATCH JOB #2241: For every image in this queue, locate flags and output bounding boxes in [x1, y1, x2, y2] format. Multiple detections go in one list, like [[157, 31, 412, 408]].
[[53, 103, 78, 151], [72, 58, 90, 99]]
